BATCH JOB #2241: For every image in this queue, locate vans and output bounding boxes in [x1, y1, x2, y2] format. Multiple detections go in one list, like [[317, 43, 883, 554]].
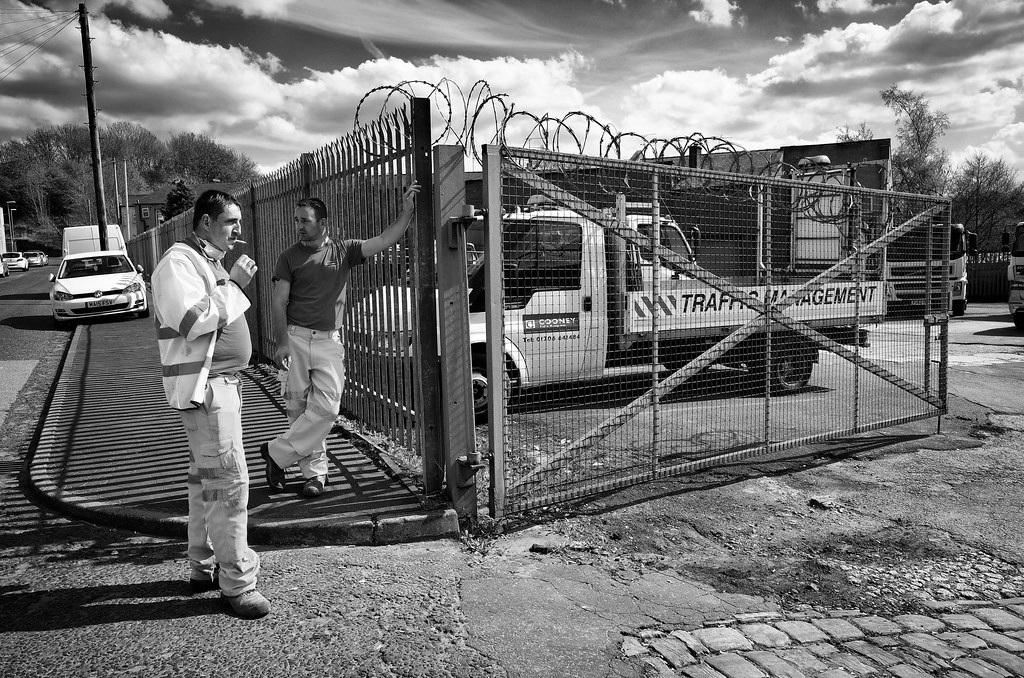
[[62, 225, 128, 259]]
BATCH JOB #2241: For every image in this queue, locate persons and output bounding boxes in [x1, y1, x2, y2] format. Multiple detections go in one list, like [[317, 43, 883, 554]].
[[150, 189, 271, 620], [259, 180, 422, 496]]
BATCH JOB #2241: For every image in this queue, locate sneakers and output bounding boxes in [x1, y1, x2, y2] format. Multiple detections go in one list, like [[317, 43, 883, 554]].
[[189, 565, 221, 594], [219, 590, 271, 620], [260, 442, 287, 490], [299, 475, 330, 498]]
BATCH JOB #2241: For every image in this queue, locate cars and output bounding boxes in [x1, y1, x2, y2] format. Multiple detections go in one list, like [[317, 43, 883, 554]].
[[49, 250, 151, 329], [39, 252, 49, 266], [23, 252, 44, 267], [0, 254, 10, 278], [2, 252, 29, 271]]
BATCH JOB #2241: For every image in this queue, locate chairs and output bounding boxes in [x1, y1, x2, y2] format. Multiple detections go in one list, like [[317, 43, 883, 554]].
[[106, 257, 125, 274], [67, 262, 89, 277]]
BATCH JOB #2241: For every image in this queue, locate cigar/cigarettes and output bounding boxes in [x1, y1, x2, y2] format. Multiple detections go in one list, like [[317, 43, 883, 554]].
[[280, 370, 288, 376], [235, 239, 247, 244]]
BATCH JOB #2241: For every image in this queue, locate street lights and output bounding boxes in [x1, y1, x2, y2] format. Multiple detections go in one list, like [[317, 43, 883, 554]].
[[6, 201, 18, 252]]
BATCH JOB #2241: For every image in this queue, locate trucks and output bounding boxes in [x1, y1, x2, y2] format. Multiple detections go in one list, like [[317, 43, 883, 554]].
[[340, 205, 890, 423], [1003, 221, 1024, 331], [887, 223, 979, 319], [624, 208, 700, 282]]
